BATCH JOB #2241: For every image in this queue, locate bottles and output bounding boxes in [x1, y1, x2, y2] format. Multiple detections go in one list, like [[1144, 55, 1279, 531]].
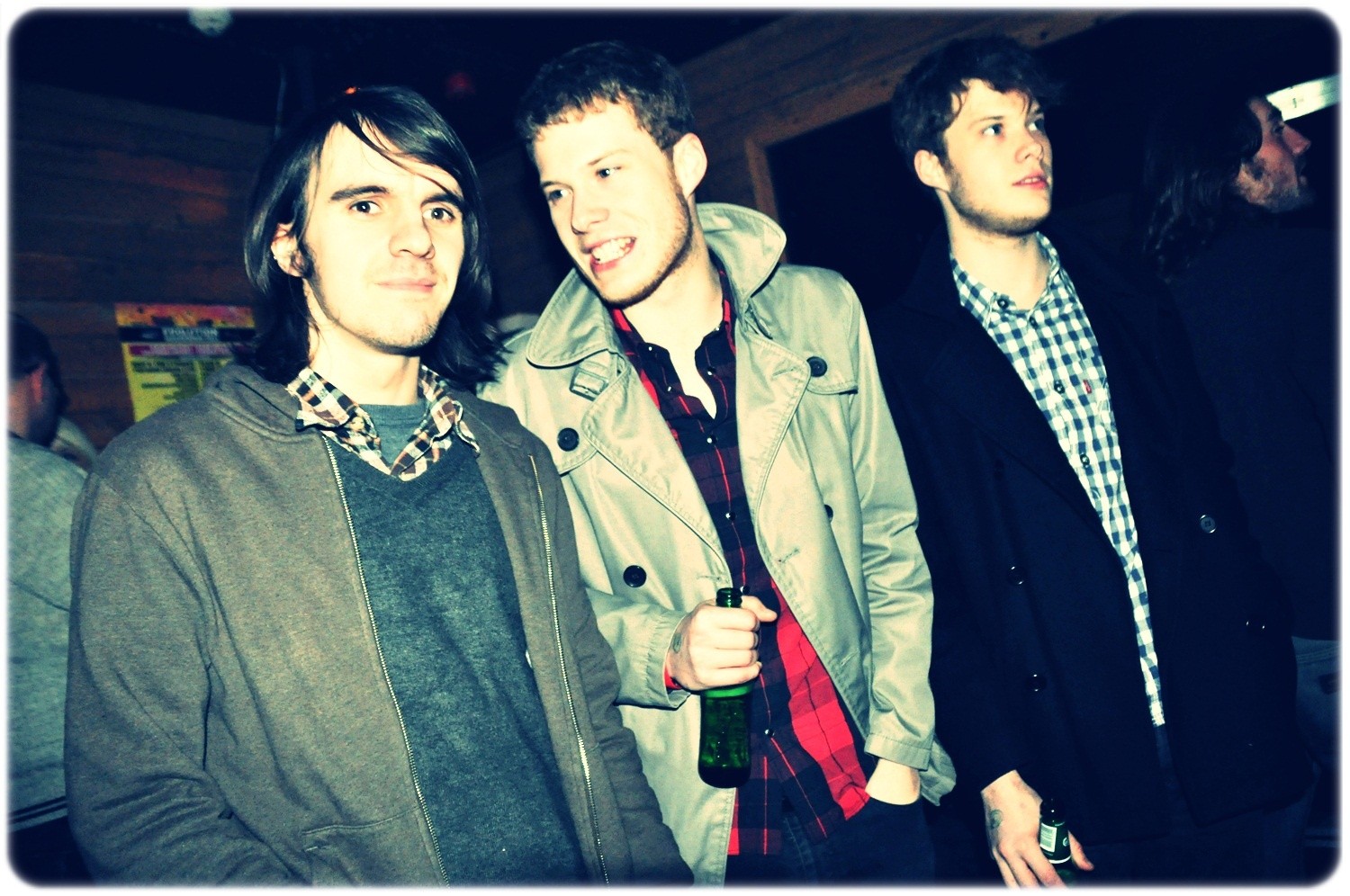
[[698, 588, 755, 788], [1027, 797, 1082, 889]]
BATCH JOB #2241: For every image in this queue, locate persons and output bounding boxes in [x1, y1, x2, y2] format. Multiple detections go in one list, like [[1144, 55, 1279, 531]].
[[10, 35, 1341, 889], [63, 78, 708, 888]]
